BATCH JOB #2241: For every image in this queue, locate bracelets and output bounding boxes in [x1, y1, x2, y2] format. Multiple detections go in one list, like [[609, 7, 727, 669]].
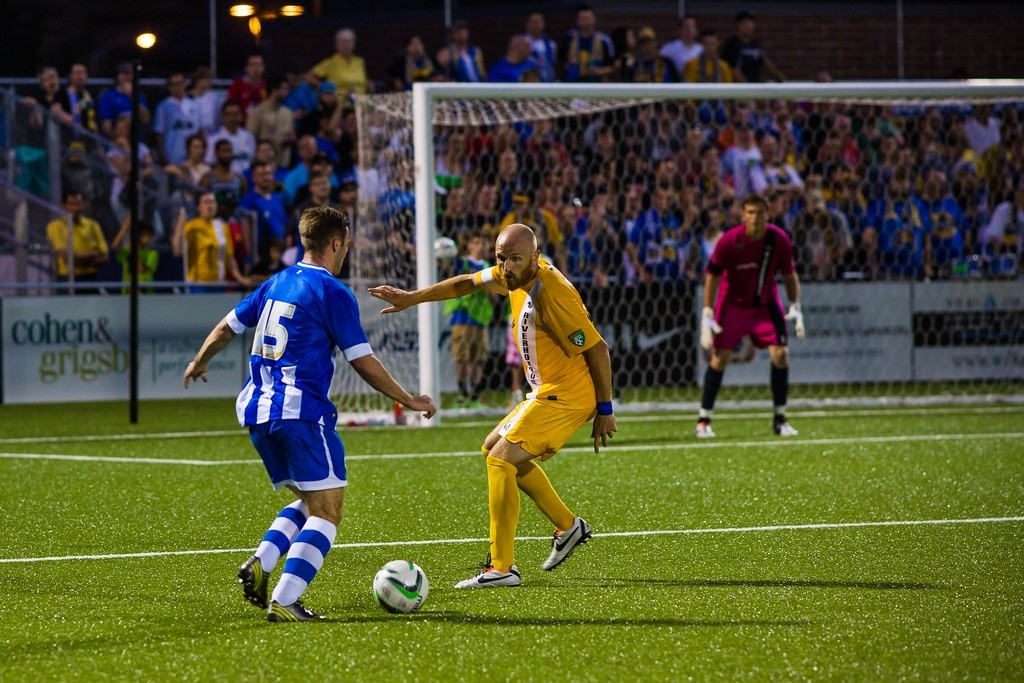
[[596, 402, 613, 415]]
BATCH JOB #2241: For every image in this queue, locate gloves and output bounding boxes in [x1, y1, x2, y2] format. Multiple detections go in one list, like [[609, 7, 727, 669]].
[[700, 307, 723, 350], [784, 304, 804, 339]]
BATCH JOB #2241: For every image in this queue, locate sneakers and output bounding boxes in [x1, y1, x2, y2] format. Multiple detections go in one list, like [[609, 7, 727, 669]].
[[543, 515, 593, 571], [454, 553, 521, 589], [237, 555, 270, 610], [697, 423, 715, 439], [774, 421, 799, 438], [267, 598, 326, 624]]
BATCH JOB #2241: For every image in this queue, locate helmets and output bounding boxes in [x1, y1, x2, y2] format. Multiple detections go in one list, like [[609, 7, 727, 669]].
[[434, 239, 458, 259]]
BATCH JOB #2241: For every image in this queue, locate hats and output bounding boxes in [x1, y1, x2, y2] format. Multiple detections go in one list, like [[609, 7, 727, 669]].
[[116, 63, 133, 72], [321, 83, 337, 92], [192, 67, 214, 78], [639, 27, 658, 41], [735, 10, 755, 24]]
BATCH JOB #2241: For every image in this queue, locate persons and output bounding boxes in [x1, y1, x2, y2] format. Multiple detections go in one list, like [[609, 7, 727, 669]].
[[0, 31, 434, 295], [368, 223, 618, 589], [422, 9, 1024, 409], [183, 205, 437, 623], [696, 196, 805, 439]]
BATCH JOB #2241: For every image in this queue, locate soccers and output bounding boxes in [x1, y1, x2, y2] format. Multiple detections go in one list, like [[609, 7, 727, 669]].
[[372, 559, 430, 614]]
